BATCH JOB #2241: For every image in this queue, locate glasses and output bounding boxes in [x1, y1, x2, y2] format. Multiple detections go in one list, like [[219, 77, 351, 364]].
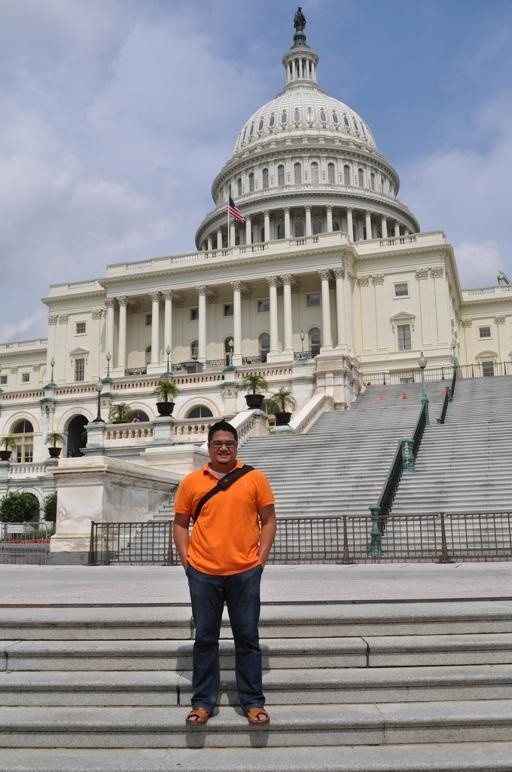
[[208, 440, 236, 447]]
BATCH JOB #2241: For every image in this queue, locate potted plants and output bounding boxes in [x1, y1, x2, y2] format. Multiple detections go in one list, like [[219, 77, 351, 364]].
[[151, 378, 181, 416], [237, 373, 297, 425], [44, 433, 65, 457], [108, 402, 132, 424], [0, 436, 16, 460]]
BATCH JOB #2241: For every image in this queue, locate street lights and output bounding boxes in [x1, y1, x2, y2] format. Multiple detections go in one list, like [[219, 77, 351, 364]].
[[50, 357, 55, 382], [228, 338, 234, 366], [106, 351, 112, 377], [449, 337, 457, 365], [92, 379, 106, 418], [165, 344, 174, 374], [417, 351, 427, 396], [300, 329, 305, 353]]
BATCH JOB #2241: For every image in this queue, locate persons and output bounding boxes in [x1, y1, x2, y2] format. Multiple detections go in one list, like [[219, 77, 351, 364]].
[[173, 421, 277, 724], [131, 415, 141, 423]]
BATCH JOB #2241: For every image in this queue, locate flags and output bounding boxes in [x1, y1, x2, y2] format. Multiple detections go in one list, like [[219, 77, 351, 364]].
[[229, 197, 243, 222]]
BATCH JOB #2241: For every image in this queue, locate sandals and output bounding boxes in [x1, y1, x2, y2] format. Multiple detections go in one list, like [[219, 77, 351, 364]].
[[186, 708, 209, 724], [247, 706, 269, 725]]
